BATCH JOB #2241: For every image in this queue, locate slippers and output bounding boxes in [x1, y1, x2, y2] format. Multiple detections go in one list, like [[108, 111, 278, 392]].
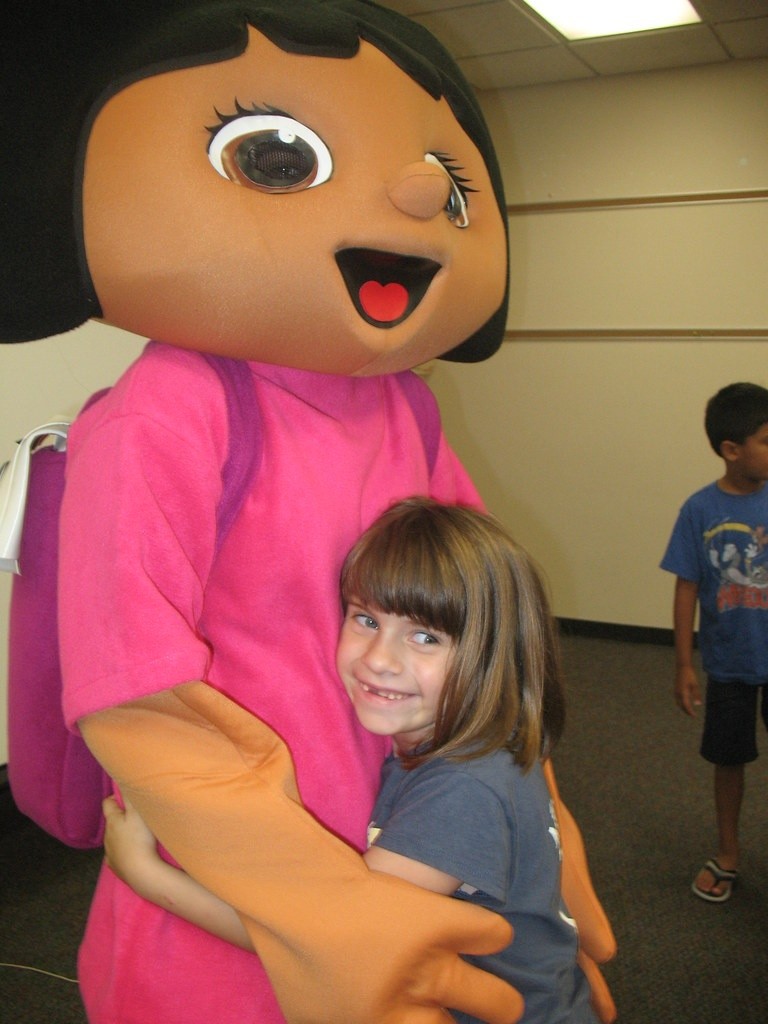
[[692, 858, 737, 902]]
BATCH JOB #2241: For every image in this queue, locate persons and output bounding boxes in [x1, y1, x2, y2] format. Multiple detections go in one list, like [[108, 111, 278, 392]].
[[661, 383, 768, 902], [104, 498, 599, 1023], [0, 0, 617, 1024]]
